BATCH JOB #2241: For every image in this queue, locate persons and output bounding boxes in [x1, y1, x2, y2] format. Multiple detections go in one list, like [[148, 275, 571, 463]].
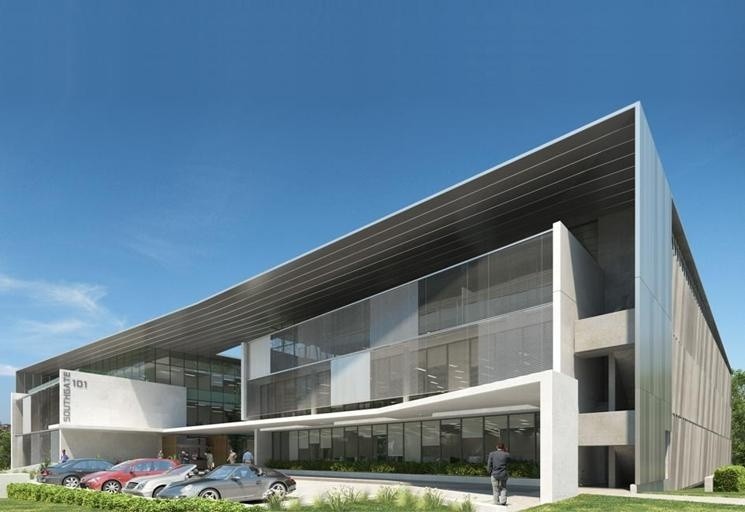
[[204, 449, 214, 471], [225, 448, 237, 464], [155, 449, 199, 466], [60, 448, 70, 464], [242, 448, 253, 464], [487, 442, 513, 506]]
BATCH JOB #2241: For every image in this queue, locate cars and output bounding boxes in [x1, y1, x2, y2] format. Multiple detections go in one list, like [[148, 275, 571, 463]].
[[39, 456, 296, 504]]
